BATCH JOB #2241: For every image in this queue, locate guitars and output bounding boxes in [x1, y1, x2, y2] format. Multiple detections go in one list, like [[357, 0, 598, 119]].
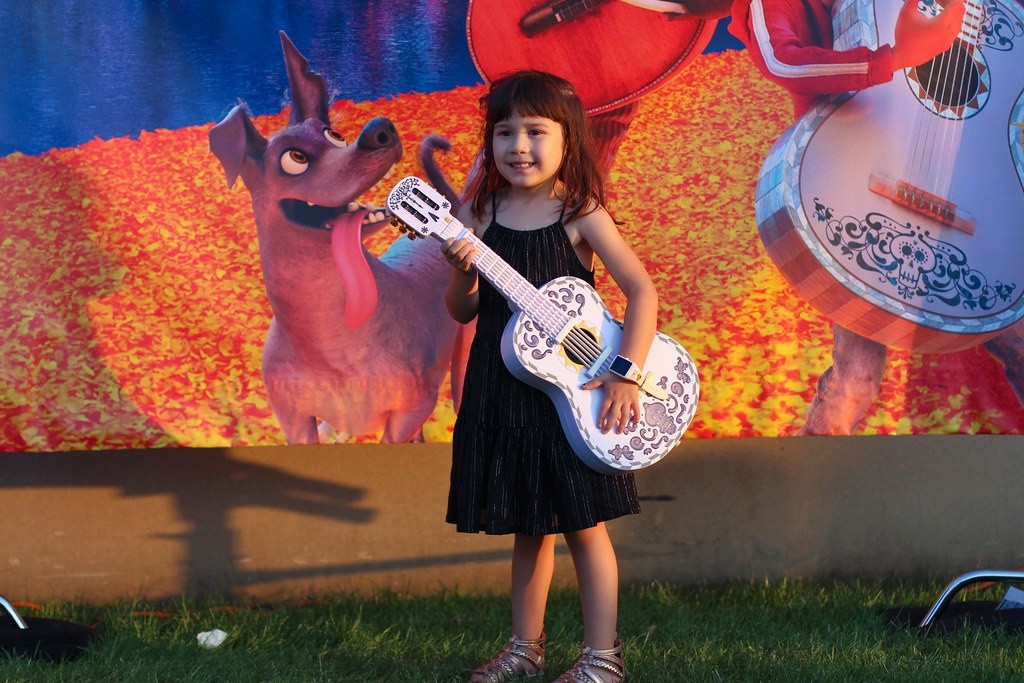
[[752, 1, 1023, 356], [386, 175, 701, 476]]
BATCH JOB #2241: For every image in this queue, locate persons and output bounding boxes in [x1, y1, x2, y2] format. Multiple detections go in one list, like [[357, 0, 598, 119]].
[[440, 70, 658, 683]]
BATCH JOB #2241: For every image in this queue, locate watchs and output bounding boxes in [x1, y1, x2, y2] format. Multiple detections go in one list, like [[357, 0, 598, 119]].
[[609, 355, 669, 400]]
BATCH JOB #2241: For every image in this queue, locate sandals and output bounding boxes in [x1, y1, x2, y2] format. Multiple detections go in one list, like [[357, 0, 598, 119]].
[[552, 641, 626, 683], [469, 632, 548, 683]]
